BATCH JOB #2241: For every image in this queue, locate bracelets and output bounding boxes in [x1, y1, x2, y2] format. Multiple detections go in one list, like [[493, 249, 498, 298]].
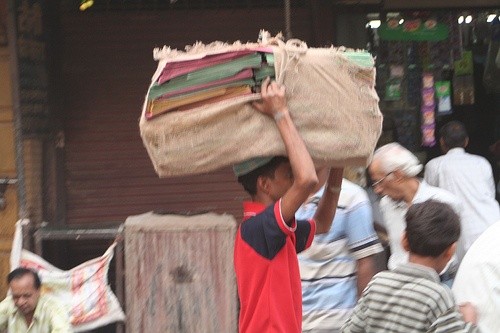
[[274, 109, 289, 120], [324, 183, 341, 193]]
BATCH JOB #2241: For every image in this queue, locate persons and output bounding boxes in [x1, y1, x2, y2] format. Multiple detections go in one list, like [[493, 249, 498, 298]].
[[295, 120, 500, 333], [232, 77, 344, 333], [0, 267, 73, 333]]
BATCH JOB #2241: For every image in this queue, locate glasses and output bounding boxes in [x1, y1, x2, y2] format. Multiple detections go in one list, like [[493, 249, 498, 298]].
[[371, 170, 406, 189]]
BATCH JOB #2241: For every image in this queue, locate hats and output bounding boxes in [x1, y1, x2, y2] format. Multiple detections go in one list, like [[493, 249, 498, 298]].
[[233, 155, 273, 177]]
